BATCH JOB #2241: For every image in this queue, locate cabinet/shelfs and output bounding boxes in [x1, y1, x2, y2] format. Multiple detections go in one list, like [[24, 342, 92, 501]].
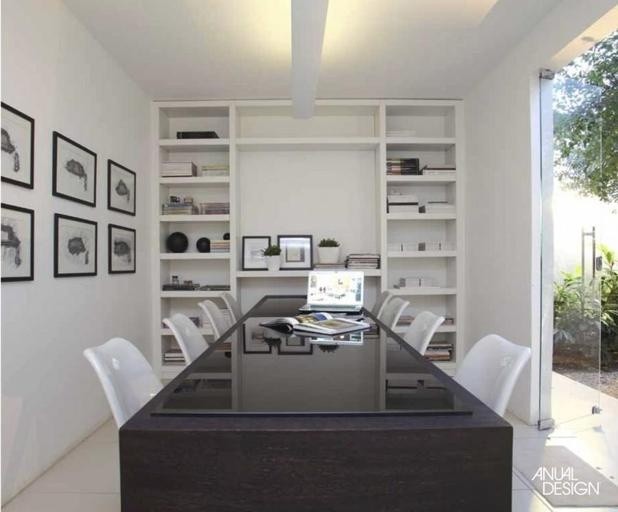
[[148, 99, 464, 375]]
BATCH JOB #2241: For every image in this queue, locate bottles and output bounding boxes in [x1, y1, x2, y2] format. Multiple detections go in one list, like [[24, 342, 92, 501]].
[[172, 276, 180, 289]]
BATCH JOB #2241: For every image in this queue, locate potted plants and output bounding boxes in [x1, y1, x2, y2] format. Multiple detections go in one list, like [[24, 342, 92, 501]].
[[317, 239, 342, 265], [265, 246, 282, 271]]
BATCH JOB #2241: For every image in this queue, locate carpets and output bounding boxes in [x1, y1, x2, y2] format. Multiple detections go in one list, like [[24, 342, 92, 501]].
[[514, 445, 618, 512]]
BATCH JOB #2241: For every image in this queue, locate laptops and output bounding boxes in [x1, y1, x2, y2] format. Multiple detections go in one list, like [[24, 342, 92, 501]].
[[309, 331, 363, 345], [297, 271, 364, 313]]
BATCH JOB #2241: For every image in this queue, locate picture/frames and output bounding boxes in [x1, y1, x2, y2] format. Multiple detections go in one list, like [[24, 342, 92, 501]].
[[52, 131, 98, 208], [278, 234, 312, 269], [106, 159, 137, 216], [107, 223, 137, 273], [55, 212, 98, 277], [241, 235, 270, 270], [1, 100, 34, 187], [1, 201, 34, 282]]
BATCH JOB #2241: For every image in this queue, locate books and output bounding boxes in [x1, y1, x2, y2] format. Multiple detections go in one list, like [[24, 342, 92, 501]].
[[259, 310, 373, 337], [384, 150, 455, 363], [155, 155, 229, 291]]
